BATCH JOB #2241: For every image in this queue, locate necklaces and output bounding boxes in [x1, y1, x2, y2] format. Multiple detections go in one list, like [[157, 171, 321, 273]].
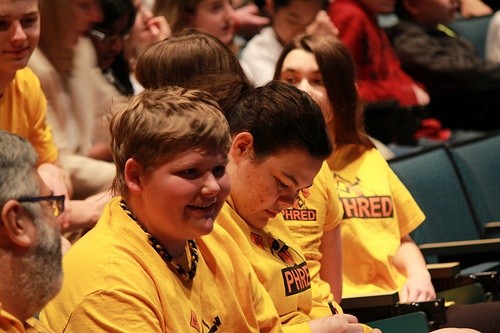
[[120, 199, 201, 284]]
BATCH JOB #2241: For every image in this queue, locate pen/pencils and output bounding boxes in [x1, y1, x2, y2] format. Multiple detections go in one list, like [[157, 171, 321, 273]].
[[327, 301, 338, 316]]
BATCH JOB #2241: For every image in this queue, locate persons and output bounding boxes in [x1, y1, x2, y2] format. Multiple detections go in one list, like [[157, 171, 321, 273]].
[[0, 0, 173, 232], [173, 0, 499, 141], [27, 85, 286, 333], [273, 32, 500, 333], [58, 26, 343, 333], [0, 130, 70, 333], [181, 70, 484, 333]]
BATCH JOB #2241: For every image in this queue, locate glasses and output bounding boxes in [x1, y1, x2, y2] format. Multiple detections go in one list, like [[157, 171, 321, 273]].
[[16, 193, 67, 217]]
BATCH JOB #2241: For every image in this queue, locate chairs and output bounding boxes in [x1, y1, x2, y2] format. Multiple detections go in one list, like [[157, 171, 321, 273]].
[[341, 16, 500, 333]]
